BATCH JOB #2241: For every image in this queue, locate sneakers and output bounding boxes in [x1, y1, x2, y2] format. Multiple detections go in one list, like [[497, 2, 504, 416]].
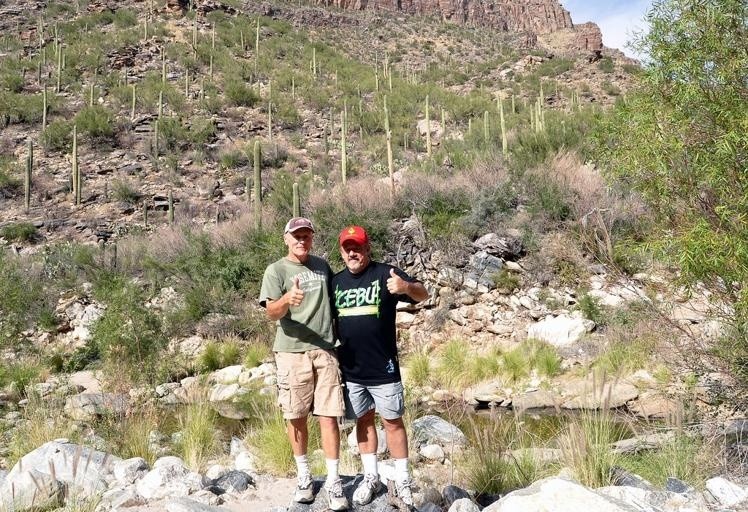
[[353, 476, 381, 504], [395, 474, 412, 510], [296, 475, 316, 503], [324, 480, 349, 511]]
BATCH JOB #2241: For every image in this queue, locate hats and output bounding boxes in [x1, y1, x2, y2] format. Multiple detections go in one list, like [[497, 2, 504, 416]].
[[339, 226, 368, 247], [284, 217, 315, 234]]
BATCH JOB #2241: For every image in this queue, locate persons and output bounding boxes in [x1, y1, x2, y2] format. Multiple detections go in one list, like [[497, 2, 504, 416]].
[[259, 216, 352, 512], [330, 224, 429, 507]]
